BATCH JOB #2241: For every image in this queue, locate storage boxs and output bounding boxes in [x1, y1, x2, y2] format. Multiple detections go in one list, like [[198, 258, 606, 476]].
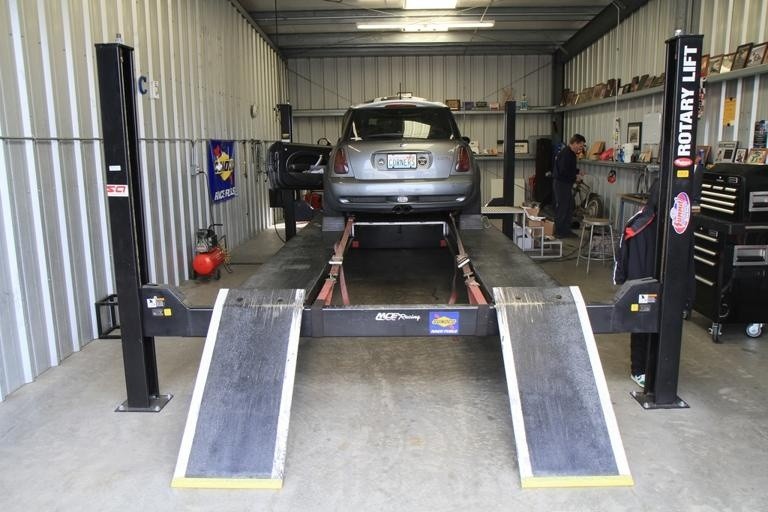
[[512, 207, 558, 250]]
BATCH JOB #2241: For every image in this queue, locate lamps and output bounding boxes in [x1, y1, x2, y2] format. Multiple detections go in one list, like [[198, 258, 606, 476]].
[[352, 20, 496, 35]]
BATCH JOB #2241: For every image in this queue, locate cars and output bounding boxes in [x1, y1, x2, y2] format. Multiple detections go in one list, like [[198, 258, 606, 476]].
[[265, 83, 481, 217]]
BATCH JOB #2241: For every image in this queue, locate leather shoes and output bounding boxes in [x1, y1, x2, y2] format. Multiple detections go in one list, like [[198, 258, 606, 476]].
[[557, 231, 578, 239]]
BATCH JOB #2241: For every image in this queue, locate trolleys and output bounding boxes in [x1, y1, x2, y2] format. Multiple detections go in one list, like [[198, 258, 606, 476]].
[[188, 223, 232, 283], [682, 162, 767, 340]]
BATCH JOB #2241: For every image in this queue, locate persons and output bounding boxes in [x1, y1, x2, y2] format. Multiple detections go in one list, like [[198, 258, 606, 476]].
[[553, 134, 585, 239]]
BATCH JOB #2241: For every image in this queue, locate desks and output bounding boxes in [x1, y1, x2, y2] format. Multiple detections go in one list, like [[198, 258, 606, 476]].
[[613, 192, 700, 247]]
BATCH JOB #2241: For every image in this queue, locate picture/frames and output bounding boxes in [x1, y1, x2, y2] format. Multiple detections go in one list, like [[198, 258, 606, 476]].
[[698, 41, 767, 77]]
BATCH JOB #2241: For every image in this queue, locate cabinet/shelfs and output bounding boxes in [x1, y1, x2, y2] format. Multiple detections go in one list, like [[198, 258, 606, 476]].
[[283, 61, 768, 171]]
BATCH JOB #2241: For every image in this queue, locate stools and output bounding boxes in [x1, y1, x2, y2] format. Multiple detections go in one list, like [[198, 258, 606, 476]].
[[574, 216, 616, 274]]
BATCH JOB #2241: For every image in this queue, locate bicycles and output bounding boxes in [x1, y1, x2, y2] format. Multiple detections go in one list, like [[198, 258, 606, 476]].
[[570, 178, 602, 230]]
